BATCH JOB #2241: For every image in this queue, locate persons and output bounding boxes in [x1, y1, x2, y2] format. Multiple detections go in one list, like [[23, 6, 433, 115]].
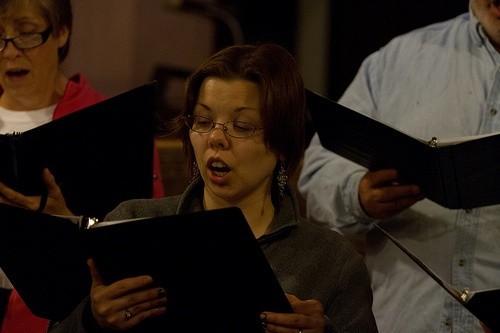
[[48, 42, 380, 333], [297, 0, 500, 333], [0, 0, 166, 333]]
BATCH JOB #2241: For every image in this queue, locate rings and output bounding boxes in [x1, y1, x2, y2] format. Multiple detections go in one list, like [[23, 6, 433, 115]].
[[299, 330, 302, 333], [124, 308, 133, 320]]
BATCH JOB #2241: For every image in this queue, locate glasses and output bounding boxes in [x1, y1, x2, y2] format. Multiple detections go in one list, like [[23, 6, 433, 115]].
[[0, 25, 54, 52], [182, 114, 263, 139]]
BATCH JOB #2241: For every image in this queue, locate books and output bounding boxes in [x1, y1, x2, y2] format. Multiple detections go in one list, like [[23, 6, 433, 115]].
[[1, 80, 294, 333], [301, 83, 500, 210]]
